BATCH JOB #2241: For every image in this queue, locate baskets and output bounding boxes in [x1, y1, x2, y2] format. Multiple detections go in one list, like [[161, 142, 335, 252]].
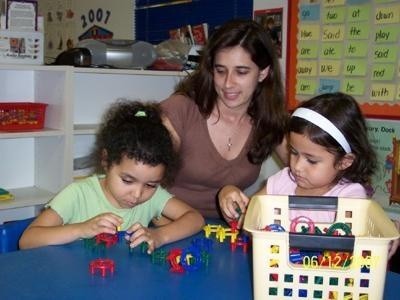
[[243, 193, 399, 299], [0, 102, 47, 131]]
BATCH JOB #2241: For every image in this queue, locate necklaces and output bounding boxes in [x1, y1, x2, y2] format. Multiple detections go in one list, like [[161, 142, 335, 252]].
[[212, 116, 248, 152]]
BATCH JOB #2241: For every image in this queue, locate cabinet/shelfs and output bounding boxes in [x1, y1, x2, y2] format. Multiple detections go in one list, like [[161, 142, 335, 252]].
[[0, 64, 72, 225], [71, 68, 194, 179]]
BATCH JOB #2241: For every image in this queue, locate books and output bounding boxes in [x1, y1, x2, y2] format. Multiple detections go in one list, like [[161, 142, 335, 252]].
[[169, 22, 209, 48]]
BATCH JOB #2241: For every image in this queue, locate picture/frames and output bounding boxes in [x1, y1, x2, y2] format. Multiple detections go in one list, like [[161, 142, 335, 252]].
[[254, 8, 283, 61]]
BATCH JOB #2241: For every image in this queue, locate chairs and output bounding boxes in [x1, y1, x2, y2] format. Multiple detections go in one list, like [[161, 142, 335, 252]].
[[0, 215, 40, 253]]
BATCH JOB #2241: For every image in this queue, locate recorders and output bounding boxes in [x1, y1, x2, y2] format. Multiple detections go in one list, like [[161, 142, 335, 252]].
[[76, 39, 156, 70]]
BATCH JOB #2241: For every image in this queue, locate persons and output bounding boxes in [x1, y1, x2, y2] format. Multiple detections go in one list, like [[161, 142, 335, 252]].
[[217, 91, 399, 262], [18, 97, 205, 256], [152, 17, 292, 226], [266, 18, 279, 51]]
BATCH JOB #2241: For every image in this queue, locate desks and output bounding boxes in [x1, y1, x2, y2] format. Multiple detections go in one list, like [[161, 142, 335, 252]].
[[1, 218, 400, 300]]
